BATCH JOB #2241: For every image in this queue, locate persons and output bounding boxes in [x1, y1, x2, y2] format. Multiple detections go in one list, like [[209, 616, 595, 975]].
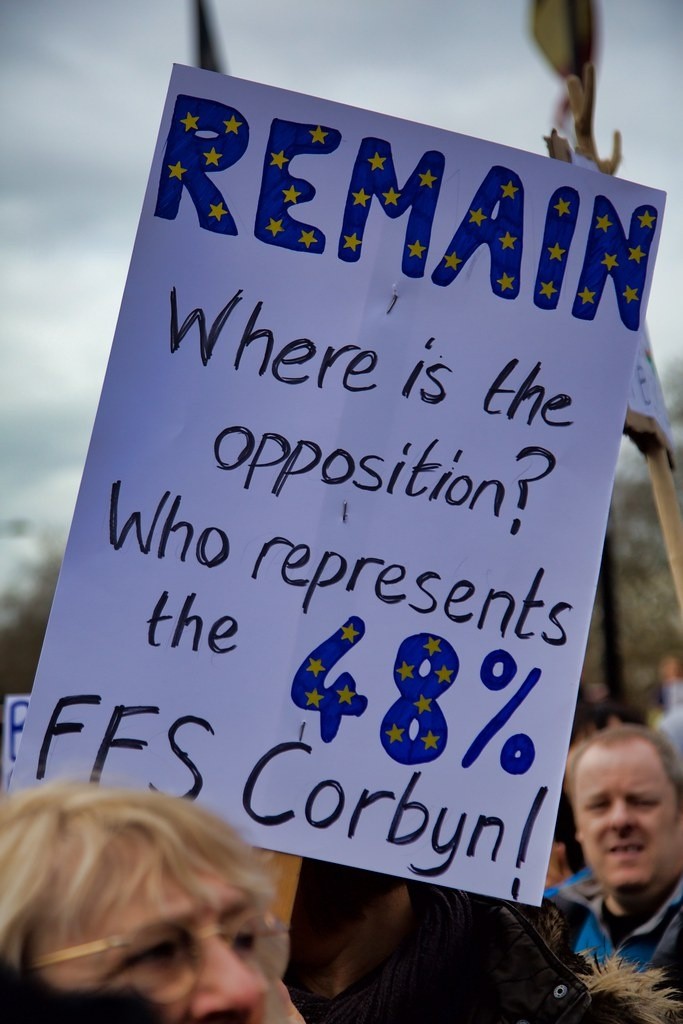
[[549, 726, 682, 994], [1, 785, 301, 1024], [285, 857, 683, 1024]]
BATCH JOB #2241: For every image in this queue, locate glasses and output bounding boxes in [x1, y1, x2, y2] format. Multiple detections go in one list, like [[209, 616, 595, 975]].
[[23, 910, 294, 1007]]
